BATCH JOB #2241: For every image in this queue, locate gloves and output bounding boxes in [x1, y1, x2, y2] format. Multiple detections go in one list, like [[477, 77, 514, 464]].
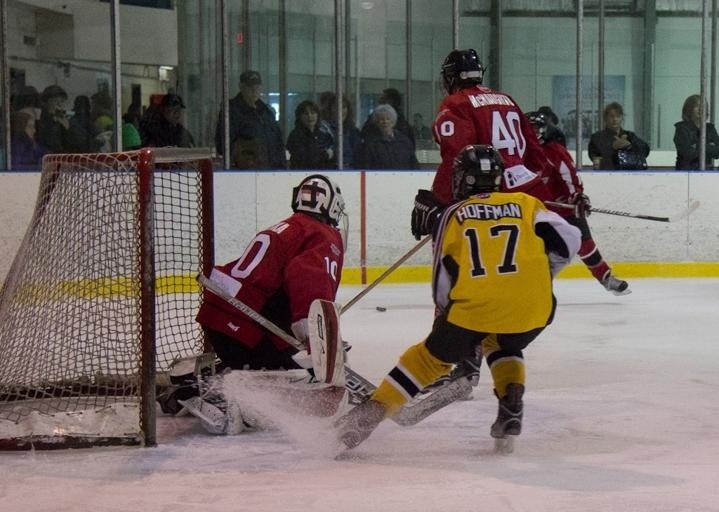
[[573, 193, 592, 220], [411, 189, 438, 241]]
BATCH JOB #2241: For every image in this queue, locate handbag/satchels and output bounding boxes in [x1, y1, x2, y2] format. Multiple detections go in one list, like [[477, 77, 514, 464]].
[[614, 138, 648, 170]]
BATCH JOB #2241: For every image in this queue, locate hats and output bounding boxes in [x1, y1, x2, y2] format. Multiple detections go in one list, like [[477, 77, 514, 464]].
[[160, 91, 186, 108], [240, 70, 261, 85]]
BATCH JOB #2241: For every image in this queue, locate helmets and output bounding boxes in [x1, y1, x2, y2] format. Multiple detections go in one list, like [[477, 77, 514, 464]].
[[291, 174, 346, 224], [439, 49, 483, 96], [451, 144, 504, 201], [524, 111, 548, 144]]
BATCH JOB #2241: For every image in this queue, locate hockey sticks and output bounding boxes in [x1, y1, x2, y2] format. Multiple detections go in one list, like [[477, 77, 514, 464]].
[[195, 272, 472, 427], [541, 201, 701, 224]]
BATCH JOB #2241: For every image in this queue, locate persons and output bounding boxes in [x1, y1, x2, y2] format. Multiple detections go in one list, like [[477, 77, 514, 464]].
[[215, 71, 433, 171], [429, 48, 556, 215], [524, 112, 628, 293], [672, 94, 719, 171], [589, 102, 650, 171], [10, 85, 195, 170], [326, 143, 582, 457], [155, 174, 347, 432]]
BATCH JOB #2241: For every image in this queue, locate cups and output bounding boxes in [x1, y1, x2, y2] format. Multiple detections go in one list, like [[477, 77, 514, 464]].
[[711, 156, 719, 170], [592, 156, 603, 170]]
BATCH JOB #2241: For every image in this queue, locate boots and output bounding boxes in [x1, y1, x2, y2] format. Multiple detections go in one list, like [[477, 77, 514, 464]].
[[333, 400, 385, 449], [600, 269, 628, 292], [490, 383, 524, 438]]
